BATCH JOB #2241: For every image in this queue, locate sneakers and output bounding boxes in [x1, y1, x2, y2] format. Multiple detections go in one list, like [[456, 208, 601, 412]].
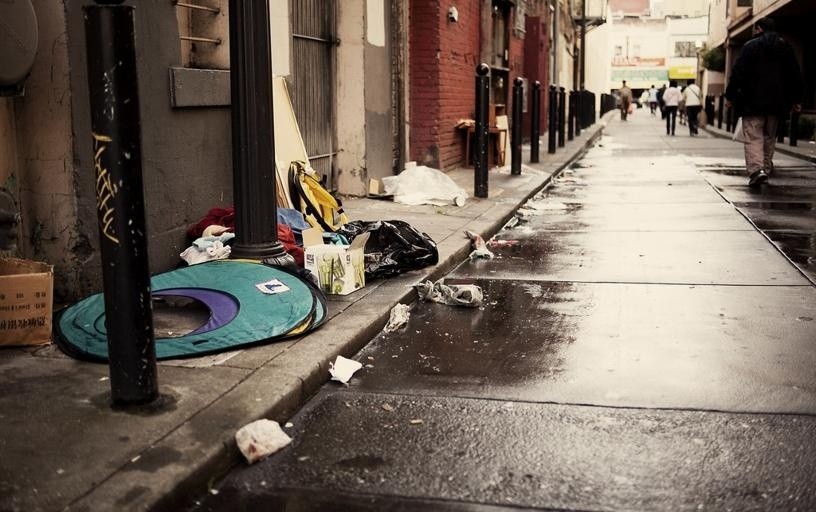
[[749, 169, 768, 187]]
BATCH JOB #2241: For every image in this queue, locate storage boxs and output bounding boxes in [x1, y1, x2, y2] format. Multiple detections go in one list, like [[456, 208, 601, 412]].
[[1, 256, 55, 348]]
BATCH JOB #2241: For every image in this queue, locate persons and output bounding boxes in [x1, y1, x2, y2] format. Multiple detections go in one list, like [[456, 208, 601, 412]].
[[641, 87, 650, 112], [680, 86, 689, 127], [725, 16, 803, 189], [663, 79, 683, 137], [647, 84, 659, 116], [618, 80, 633, 120], [656, 83, 669, 118], [683, 77, 703, 139]]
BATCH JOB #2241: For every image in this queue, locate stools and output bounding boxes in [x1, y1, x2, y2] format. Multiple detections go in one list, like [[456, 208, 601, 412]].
[[465, 127, 507, 167]]
